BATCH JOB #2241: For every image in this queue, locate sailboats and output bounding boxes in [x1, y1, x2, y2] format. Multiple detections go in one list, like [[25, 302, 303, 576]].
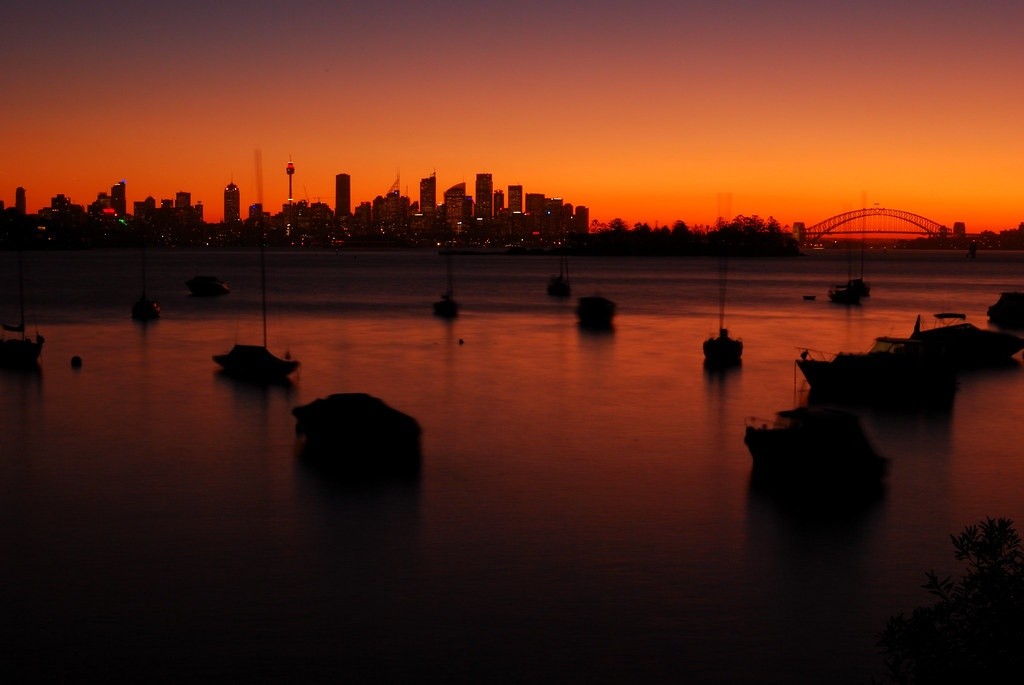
[[546, 232, 572, 298], [826, 224, 870, 306], [432, 274, 460, 319], [702, 252, 744, 371], [132, 270, 162, 320], [0, 229, 44, 370], [209, 149, 300, 387]]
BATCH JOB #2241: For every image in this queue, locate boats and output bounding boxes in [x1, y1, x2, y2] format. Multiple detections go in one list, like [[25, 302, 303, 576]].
[[802, 296, 816, 300], [291, 392, 422, 473], [186, 274, 233, 297], [576, 296, 616, 323], [968, 242, 976, 255], [438, 247, 507, 256], [742, 289, 1023, 507]]
